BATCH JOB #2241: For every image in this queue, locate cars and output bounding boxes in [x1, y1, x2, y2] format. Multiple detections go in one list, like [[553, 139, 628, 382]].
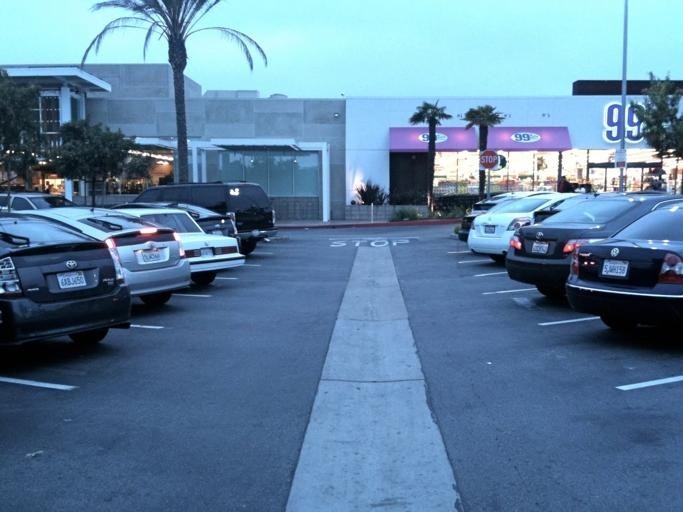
[[459, 188, 589, 264], [507, 190, 683, 306], [0, 212, 133, 359], [0, 190, 81, 212], [10, 205, 193, 309], [115, 202, 243, 249], [562, 201, 683, 343], [112, 207, 249, 290]]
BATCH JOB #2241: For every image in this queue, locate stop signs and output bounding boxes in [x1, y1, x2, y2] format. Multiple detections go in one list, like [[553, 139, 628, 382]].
[[479, 151, 498, 169]]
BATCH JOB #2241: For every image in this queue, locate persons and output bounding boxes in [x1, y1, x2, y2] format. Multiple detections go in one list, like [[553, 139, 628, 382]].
[[557, 176, 576, 193], [643, 178, 659, 191]]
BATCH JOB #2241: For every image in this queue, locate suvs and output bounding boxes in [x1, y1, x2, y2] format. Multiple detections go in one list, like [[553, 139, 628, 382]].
[[129, 180, 278, 255]]
